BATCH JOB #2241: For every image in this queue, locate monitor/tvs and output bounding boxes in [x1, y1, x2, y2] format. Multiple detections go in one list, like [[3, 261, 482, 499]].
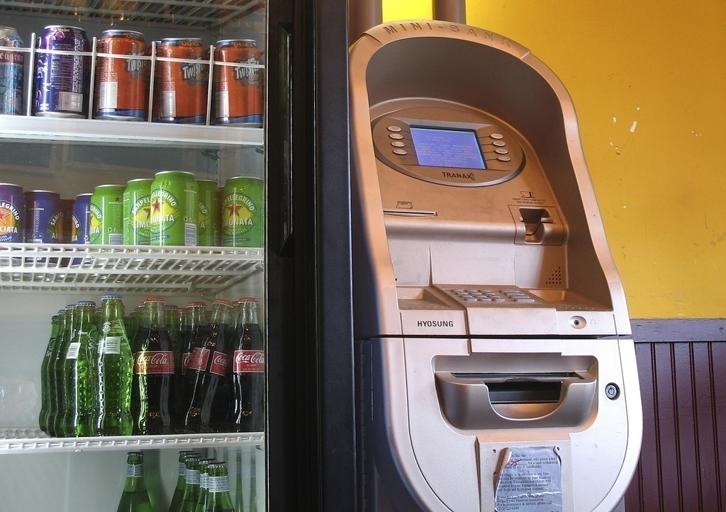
[[410, 125, 486, 171]]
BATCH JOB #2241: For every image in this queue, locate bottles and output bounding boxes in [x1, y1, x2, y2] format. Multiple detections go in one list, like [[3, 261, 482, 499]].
[[116, 450, 236, 512], [38, 293, 266, 438]]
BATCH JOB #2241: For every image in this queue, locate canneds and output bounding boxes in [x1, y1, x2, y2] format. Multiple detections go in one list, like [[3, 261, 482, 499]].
[[0, 170, 264, 267], [0, 24, 264, 129]]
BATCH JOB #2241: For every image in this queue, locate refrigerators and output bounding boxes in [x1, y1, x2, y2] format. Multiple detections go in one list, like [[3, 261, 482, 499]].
[[1, 1, 316, 512]]
